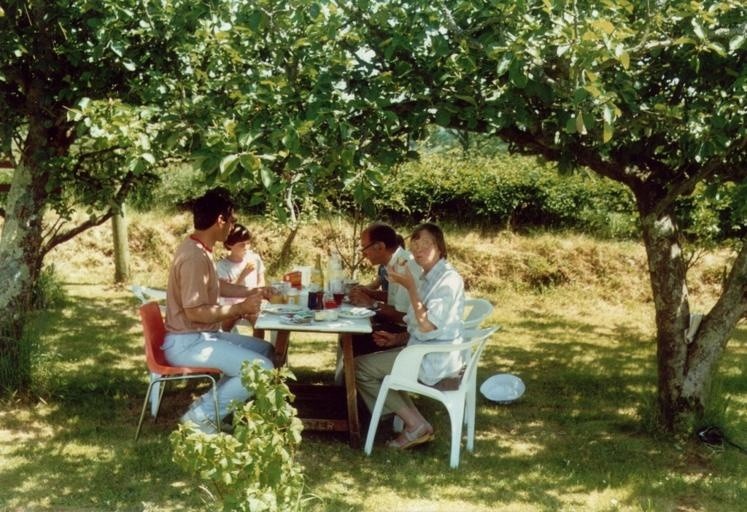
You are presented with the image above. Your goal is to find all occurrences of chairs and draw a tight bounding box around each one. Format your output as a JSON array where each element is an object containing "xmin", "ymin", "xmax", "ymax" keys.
[
  {"xmin": 363, "ymin": 324, "xmax": 501, "ymax": 470},
  {"xmin": 135, "ymin": 301, "xmax": 223, "ymax": 440},
  {"xmin": 456, "ymin": 299, "xmax": 495, "ymax": 367},
  {"xmin": 129, "ymin": 284, "xmax": 222, "ymax": 421}
]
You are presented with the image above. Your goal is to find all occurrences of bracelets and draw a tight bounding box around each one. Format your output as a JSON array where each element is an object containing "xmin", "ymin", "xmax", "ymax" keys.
[{"xmin": 398, "ymin": 332, "xmax": 408, "ymax": 345}]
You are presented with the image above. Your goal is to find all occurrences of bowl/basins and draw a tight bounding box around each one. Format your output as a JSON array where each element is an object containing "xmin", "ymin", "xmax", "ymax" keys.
[{"xmin": 271, "ymin": 281, "xmax": 291, "ymax": 293}]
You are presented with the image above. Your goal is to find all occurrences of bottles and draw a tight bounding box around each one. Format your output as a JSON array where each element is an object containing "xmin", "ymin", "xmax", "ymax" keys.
[
  {"xmin": 307, "ymin": 252, "xmax": 323, "ymax": 309},
  {"xmin": 327, "ymin": 250, "xmax": 343, "ymax": 297}
]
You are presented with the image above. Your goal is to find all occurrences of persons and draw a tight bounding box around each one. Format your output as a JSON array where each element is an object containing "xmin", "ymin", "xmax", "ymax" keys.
[
  {"xmin": 353, "ymin": 223, "xmax": 464, "ymax": 451},
  {"xmin": 347, "ymin": 224, "xmax": 423, "ymax": 442},
  {"xmin": 342, "ymin": 234, "xmax": 405, "ymax": 292},
  {"xmin": 216, "ymin": 223, "xmax": 290, "ymax": 368},
  {"xmin": 159, "ymin": 186, "xmax": 277, "ymax": 437}
]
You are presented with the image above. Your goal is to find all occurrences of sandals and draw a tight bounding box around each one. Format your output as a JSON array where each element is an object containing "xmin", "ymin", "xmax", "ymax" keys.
[{"xmin": 385, "ymin": 421, "xmax": 435, "ymax": 450}]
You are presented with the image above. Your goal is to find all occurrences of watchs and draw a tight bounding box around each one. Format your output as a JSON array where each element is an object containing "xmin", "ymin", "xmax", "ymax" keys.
[{"xmin": 371, "ymin": 300, "xmax": 384, "ymax": 309}]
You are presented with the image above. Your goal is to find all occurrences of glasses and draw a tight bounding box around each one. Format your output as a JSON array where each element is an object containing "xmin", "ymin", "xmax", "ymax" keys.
[{"xmin": 411, "ymin": 238, "xmax": 436, "ymax": 250}]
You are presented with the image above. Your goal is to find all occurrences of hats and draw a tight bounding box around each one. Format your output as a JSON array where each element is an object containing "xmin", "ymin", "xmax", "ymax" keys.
[{"xmin": 479, "ymin": 373, "xmax": 525, "ymax": 405}]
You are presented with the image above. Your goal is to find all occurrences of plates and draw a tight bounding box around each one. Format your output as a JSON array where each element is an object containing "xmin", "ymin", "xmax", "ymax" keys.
[
  {"xmin": 262, "ymin": 303, "xmax": 303, "ymax": 314},
  {"xmin": 332, "ymin": 307, "xmax": 374, "ymax": 319}
]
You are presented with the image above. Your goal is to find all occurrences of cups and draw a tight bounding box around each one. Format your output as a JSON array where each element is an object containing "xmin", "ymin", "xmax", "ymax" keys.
[
  {"xmin": 269, "ymin": 294, "xmax": 299, "ymax": 305},
  {"xmin": 282, "ymin": 271, "xmax": 302, "ymax": 290},
  {"xmin": 324, "ymin": 291, "xmax": 344, "ymax": 309},
  {"xmin": 313, "ymin": 311, "xmax": 325, "ymax": 322}
]
